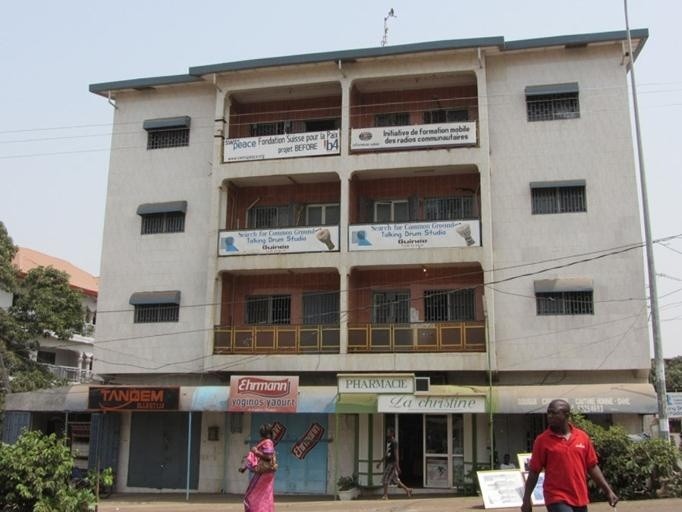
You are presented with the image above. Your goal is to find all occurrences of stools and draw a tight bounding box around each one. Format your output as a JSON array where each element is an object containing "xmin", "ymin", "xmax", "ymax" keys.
[{"xmin": 457, "ymin": 482, "xmax": 474, "ymax": 496}]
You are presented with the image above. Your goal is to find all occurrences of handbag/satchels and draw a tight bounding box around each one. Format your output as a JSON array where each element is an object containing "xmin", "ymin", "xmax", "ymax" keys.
[{"xmin": 247, "ymin": 452, "xmax": 278, "ymax": 474}]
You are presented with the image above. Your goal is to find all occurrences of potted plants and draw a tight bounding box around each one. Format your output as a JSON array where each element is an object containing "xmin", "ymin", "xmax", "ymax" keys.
[{"xmin": 335, "ymin": 475, "xmax": 361, "ymax": 501}]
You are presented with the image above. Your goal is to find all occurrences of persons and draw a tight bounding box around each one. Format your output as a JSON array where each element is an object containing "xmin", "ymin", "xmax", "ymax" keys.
[
  {"xmin": 376, "ymin": 427, "xmax": 413, "ymax": 500},
  {"xmin": 238, "ymin": 423, "xmax": 277, "ymax": 512},
  {"xmin": 520, "ymin": 399, "xmax": 619, "ymax": 512}
]
